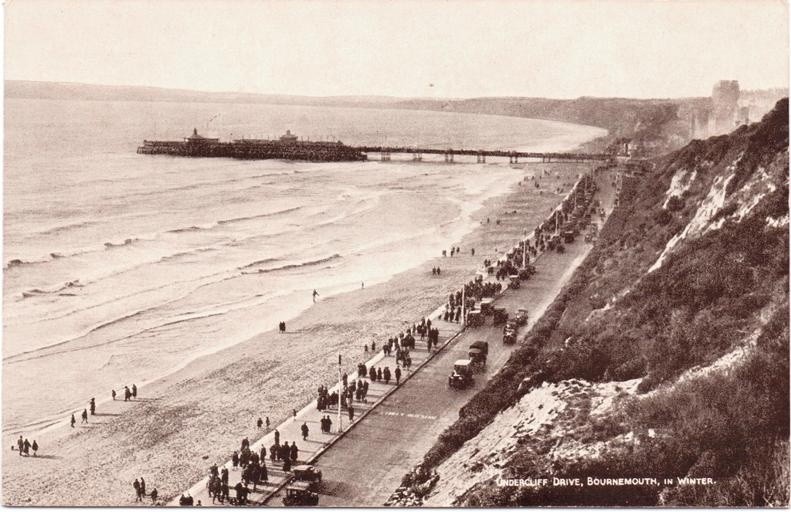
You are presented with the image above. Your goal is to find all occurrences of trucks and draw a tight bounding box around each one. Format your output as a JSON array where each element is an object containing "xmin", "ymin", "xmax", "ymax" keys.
[{"xmin": 468, "ymin": 340, "xmax": 488, "ymax": 374}]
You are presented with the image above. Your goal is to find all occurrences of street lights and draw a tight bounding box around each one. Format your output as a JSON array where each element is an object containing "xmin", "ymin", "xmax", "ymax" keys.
[{"xmin": 338, "ymin": 353, "xmax": 343, "ymax": 432}]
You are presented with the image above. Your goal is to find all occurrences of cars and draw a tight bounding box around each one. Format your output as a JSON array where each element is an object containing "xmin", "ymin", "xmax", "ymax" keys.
[
  {"xmin": 547, "ymin": 184, "xmax": 605, "ymax": 250},
  {"xmin": 510, "ymin": 265, "xmax": 536, "ymax": 289},
  {"xmin": 282, "ymin": 465, "xmax": 322, "ymax": 506},
  {"xmin": 467, "ymin": 299, "xmax": 528, "ymax": 344},
  {"xmin": 449, "ymin": 359, "xmax": 474, "ymax": 389}
]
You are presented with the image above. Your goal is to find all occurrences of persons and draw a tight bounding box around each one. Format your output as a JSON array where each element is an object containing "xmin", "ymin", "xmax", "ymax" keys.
[
  {"xmin": 148, "ymin": 486, "xmax": 158, "ymax": 504},
  {"xmin": 23, "ymin": 438, "xmax": 32, "ymax": 456},
  {"xmin": 179, "ymin": 318, "xmax": 442, "ymax": 507},
  {"xmin": 132, "ymin": 384, "xmax": 138, "ymax": 398},
  {"xmin": 81, "ymin": 408, "xmax": 89, "ymax": 424},
  {"xmin": 140, "ymin": 476, "xmax": 146, "ymax": 496},
  {"xmin": 125, "ymin": 385, "xmax": 131, "ymax": 401},
  {"xmin": 133, "ymin": 478, "xmax": 141, "ymax": 489},
  {"xmin": 17, "ymin": 435, "xmax": 24, "ymax": 455},
  {"xmin": 71, "ymin": 413, "xmax": 76, "ymax": 427},
  {"xmin": 136, "ymin": 486, "xmax": 144, "ymax": 502},
  {"xmin": 32, "ymin": 439, "xmax": 39, "ymax": 455},
  {"xmin": 90, "ymin": 397, "xmax": 96, "ymax": 414},
  {"xmin": 112, "ymin": 389, "xmax": 117, "ymax": 400},
  {"xmin": 278, "ymin": 288, "xmax": 319, "ymax": 333},
  {"xmin": 433, "ymin": 162, "xmax": 620, "ymax": 324}
]
